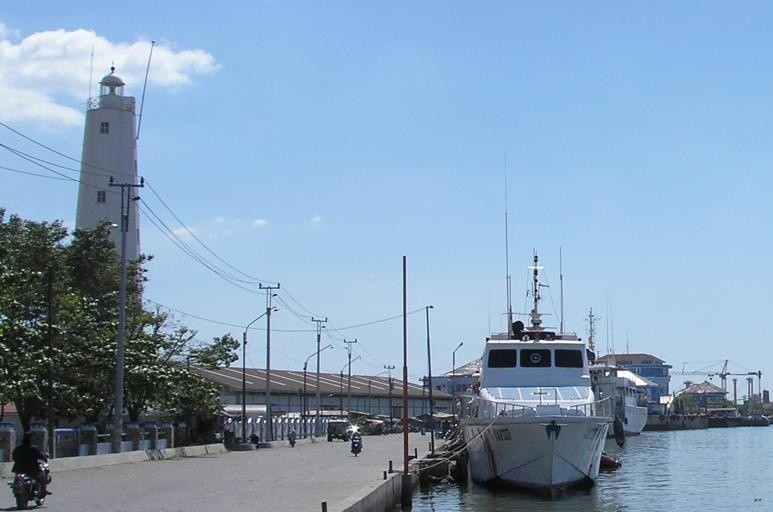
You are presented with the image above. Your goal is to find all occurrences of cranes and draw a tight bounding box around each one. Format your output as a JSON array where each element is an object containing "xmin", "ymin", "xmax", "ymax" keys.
[{"xmin": 668, "ymin": 360, "xmax": 762, "ymax": 393}]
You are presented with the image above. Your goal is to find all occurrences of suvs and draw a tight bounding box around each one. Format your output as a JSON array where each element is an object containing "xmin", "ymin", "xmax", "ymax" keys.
[{"xmin": 326, "ymin": 420, "xmax": 351, "ymax": 442}]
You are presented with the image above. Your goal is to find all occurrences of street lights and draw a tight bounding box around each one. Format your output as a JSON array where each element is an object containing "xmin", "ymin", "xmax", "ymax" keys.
[
  {"xmin": 47, "ymin": 220, "xmax": 118, "ymax": 460},
  {"xmin": 303, "ymin": 344, "xmax": 334, "ymax": 440},
  {"xmin": 452, "ymin": 342, "xmax": 463, "ymax": 375},
  {"xmin": 340, "ymin": 355, "xmax": 362, "ymax": 419},
  {"xmin": 241, "ymin": 306, "xmax": 279, "ymax": 446},
  {"xmin": 367, "ymin": 371, "xmax": 389, "ymax": 415}
]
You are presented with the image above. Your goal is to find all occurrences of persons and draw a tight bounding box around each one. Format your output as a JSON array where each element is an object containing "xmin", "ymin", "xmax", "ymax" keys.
[
  {"xmin": 351, "ymin": 429, "xmax": 362, "ymax": 452},
  {"xmin": 289, "ymin": 428, "xmax": 296, "ymax": 443},
  {"xmin": 249, "ymin": 431, "xmax": 260, "ymax": 449},
  {"xmin": 11, "ymin": 432, "xmax": 48, "ymax": 498}
]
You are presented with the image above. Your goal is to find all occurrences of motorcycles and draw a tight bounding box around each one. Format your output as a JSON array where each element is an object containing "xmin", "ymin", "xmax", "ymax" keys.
[
  {"xmin": 10, "ymin": 452, "xmax": 53, "ymax": 508},
  {"xmin": 287, "ymin": 431, "xmax": 296, "ymax": 448},
  {"xmin": 350, "ymin": 436, "xmax": 362, "ymax": 458}
]
